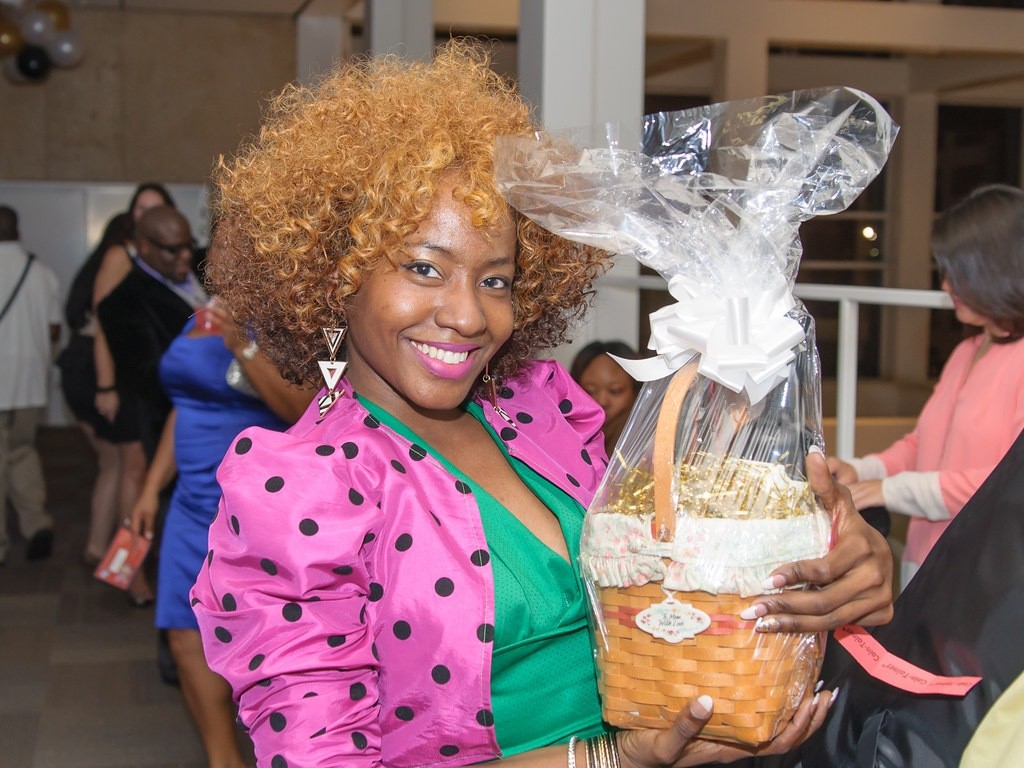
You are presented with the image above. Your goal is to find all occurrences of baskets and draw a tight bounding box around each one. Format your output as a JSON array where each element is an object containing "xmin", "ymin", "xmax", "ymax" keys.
[{"xmin": 580, "ymin": 348, "xmax": 836, "ymax": 748}]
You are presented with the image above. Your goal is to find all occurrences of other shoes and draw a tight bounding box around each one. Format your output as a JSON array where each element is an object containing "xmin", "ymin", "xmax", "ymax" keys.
[
  {"xmin": 126, "ymin": 589, "xmax": 156, "ymax": 608},
  {"xmin": 27, "ymin": 529, "xmax": 54, "ymax": 562}
]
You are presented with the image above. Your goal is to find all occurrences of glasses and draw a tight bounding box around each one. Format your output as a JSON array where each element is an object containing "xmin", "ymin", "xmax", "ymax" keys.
[{"xmin": 146, "ymin": 234, "xmax": 197, "ymax": 255}]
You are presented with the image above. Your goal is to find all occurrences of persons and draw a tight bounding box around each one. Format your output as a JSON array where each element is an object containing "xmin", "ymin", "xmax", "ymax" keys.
[
  {"xmin": 108, "ymin": 207, "xmax": 207, "ymax": 461},
  {"xmin": 566, "ymin": 339, "xmax": 638, "ymax": 456},
  {"xmin": 61, "ymin": 186, "xmax": 179, "ymax": 607},
  {"xmin": 192, "ymin": 34, "xmax": 895, "ymax": 768},
  {"xmin": 100, "ymin": 291, "xmax": 319, "ymax": 768},
  {"xmin": 0, "ymin": 204, "xmax": 62, "ymax": 565},
  {"xmin": 828, "ymin": 183, "xmax": 1023, "ymax": 598}
]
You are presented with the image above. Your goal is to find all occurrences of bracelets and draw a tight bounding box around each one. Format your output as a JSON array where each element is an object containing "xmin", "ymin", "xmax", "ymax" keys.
[
  {"xmin": 568, "ymin": 732, "xmax": 622, "ymax": 768},
  {"xmin": 96, "ymin": 384, "xmax": 116, "ymax": 393}
]
